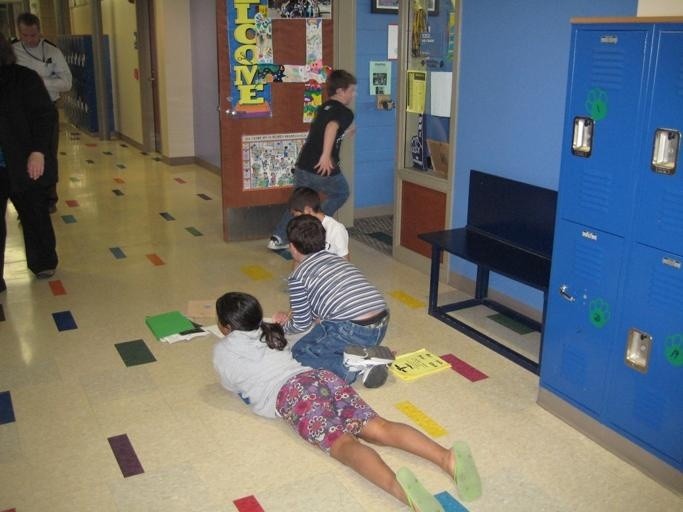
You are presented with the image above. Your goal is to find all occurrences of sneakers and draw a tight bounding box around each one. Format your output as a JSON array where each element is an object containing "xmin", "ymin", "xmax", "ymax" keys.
[
  {"xmin": 37, "ymin": 268, "xmax": 55, "ymax": 278},
  {"xmin": 344, "ymin": 343, "xmax": 397, "ymax": 388},
  {"xmin": 267, "ymin": 236, "xmax": 291, "ymax": 252}
]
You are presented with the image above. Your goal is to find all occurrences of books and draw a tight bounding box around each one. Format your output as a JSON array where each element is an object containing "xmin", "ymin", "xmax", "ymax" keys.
[{"xmin": 388, "ymin": 348, "xmax": 452, "ymax": 381}]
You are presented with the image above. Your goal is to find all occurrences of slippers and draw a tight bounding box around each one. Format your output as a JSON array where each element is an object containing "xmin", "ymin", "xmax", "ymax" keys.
[
  {"xmin": 451, "ymin": 440, "xmax": 483, "ymax": 502},
  {"xmin": 395, "ymin": 465, "xmax": 442, "ymax": 511}
]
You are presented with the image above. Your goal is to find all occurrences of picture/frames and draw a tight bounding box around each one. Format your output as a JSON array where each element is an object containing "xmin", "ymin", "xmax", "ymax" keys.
[{"xmin": 369, "ymin": 0, "xmax": 440, "ymax": 17}]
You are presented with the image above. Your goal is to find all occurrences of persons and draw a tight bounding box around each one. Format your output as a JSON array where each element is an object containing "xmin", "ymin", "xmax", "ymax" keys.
[
  {"xmin": 274, "ymin": 215, "xmax": 396, "ymax": 389},
  {"xmin": 1, "ymin": 37, "xmax": 61, "ymax": 291},
  {"xmin": 213, "ymin": 291, "xmax": 482, "ymax": 512},
  {"xmin": 266, "ymin": 69, "xmax": 357, "ymax": 250},
  {"xmin": 287, "ymin": 188, "xmax": 349, "ymax": 264},
  {"xmin": 9, "ymin": 13, "xmax": 73, "ymax": 218}
]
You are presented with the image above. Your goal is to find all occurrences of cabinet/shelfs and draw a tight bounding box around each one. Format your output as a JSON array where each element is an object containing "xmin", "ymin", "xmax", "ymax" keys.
[
  {"xmin": 557, "ymin": 16, "xmax": 653, "ymax": 242},
  {"xmin": 603, "ymin": 240, "xmax": 683, "ymax": 470},
  {"xmin": 541, "ymin": 218, "xmax": 628, "ymax": 428},
  {"xmin": 630, "ymin": 22, "xmax": 683, "ymax": 256},
  {"xmin": 56, "ymin": 33, "xmax": 116, "ymax": 139}
]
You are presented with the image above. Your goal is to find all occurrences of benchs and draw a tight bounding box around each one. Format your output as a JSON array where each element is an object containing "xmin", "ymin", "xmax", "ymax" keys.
[{"xmin": 417, "ymin": 170, "xmax": 558, "ymax": 373}]
